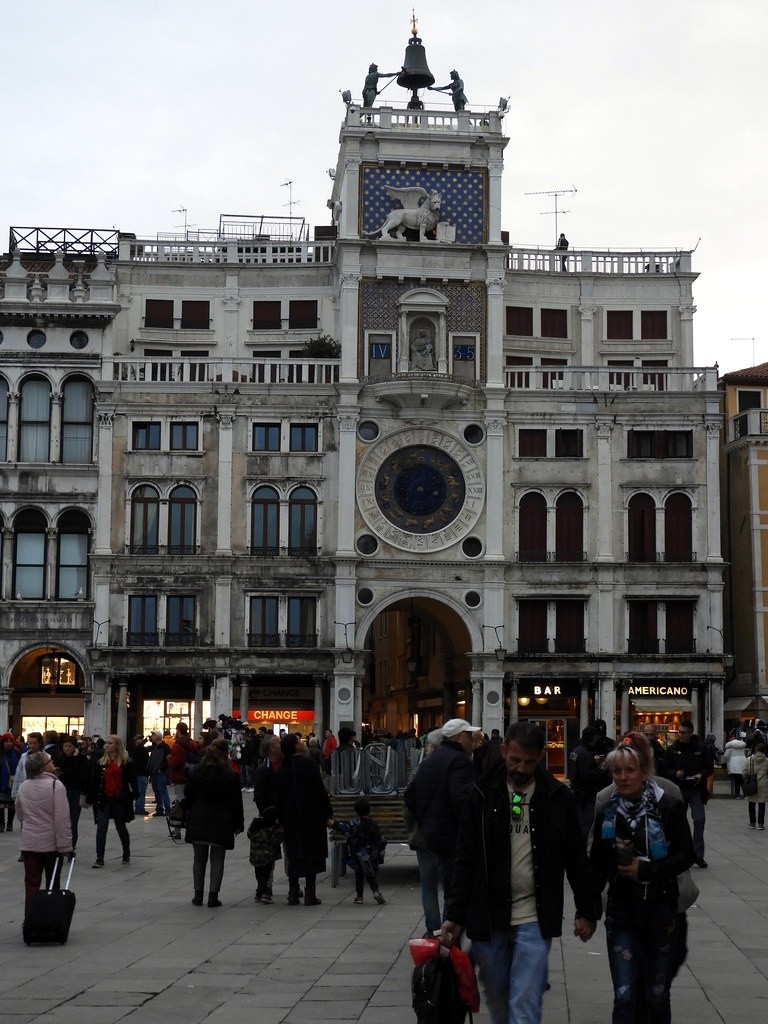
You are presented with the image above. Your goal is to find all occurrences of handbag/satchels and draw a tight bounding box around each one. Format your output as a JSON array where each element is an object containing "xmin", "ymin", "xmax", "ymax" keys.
[
  {"xmin": 79, "ymin": 794, "xmax": 89, "ymax": 809},
  {"xmin": 165, "ymin": 799, "xmax": 190, "ymax": 844},
  {"xmin": 743, "ymin": 757, "xmax": 758, "ymax": 796},
  {"xmin": 9, "ymin": 775, "xmax": 15, "ymax": 788},
  {"xmin": 678, "ymin": 871, "xmax": 699, "ymax": 914}
]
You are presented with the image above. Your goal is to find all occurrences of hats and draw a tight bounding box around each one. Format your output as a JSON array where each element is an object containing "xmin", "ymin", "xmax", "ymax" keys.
[
  {"xmin": 133, "ymin": 734, "xmax": 144, "ymax": 741},
  {"xmin": 0, "ymin": 733, "xmax": 14, "ymax": 744},
  {"xmin": 442, "ymin": 718, "xmax": 482, "ymax": 738},
  {"xmin": 151, "ymin": 731, "xmax": 162, "ymax": 739}
]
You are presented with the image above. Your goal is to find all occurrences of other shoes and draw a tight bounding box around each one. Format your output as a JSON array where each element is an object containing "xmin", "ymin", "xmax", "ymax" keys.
[
  {"xmin": 136, "ymin": 808, "xmax": 150, "ymax": 815},
  {"xmin": 92, "ymin": 858, "xmax": 104, "ymax": 868},
  {"xmin": 171, "ymin": 830, "xmax": 181, "ymax": 839},
  {"xmin": 758, "ymin": 824, "xmax": 764, "ymax": 829},
  {"xmin": 152, "ymin": 799, "xmax": 158, "ymax": 804},
  {"xmin": 695, "ymin": 858, "xmax": 708, "ymax": 868},
  {"xmin": 748, "ymin": 823, "xmax": 755, "ymax": 828},
  {"xmin": 68, "ymin": 853, "xmax": 73, "ymax": 863},
  {"xmin": 122, "ymin": 857, "xmax": 130, "ymax": 864}
]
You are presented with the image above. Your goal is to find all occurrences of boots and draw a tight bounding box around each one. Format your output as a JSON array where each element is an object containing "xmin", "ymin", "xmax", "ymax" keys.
[
  {"xmin": 7, "ymin": 808, "xmax": 15, "ymax": 831},
  {"xmin": 192, "ymin": 887, "xmax": 385, "ymax": 908},
  {"xmin": 165, "ymin": 807, "xmax": 171, "ymax": 816},
  {"xmin": 0, "ymin": 808, "xmax": 6, "ymax": 833},
  {"xmin": 152, "ymin": 807, "xmax": 164, "ymax": 817}
]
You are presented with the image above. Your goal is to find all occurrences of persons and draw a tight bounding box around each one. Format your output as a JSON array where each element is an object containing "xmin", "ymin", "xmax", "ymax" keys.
[
  {"xmin": 14, "ymin": 750, "xmax": 74, "ymax": 927},
  {"xmin": 185, "ymin": 738, "xmax": 244, "ymax": 907},
  {"xmin": 574, "ymin": 745, "xmax": 698, "ymax": 1024},
  {"xmin": 269, "ymin": 734, "xmax": 334, "ymax": 905},
  {"xmin": 360, "ymin": 63, "xmax": 401, "ymax": 126},
  {"xmin": 428, "ymin": 69, "xmax": 473, "ymax": 125},
  {"xmin": 247, "ymin": 806, "xmax": 287, "ymax": 904},
  {"xmin": 54, "ymin": 734, "xmax": 141, "ymax": 868},
  {"xmin": 438, "ymin": 722, "xmax": 602, "ymax": 1024},
  {"xmin": 0, "ymin": 715, "xmax": 768, "ymax": 833},
  {"xmin": 587, "ymin": 731, "xmax": 684, "ymax": 912},
  {"xmin": 403, "ymin": 718, "xmax": 493, "ymax": 941},
  {"xmin": 328, "ymin": 799, "xmax": 388, "ymax": 905},
  {"xmin": 554, "ymin": 233, "xmax": 569, "ymax": 272}
]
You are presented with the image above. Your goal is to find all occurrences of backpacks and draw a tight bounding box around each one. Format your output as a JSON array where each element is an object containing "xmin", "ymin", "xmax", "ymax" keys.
[
  {"xmin": 184, "ymin": 747, "xmax": 202, "ymax": 782},
  {"xmin": 410, "ymin": 936, "xmax": 480, "ymax": 1024}
]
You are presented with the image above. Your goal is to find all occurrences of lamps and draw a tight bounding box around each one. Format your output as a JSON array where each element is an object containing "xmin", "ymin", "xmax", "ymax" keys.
[
  {"xmin": 50, "ymin": 647, "xmax": 58, "ymax": 696},
  {"xmin": 483, "ymin": 625, "xmax": 507, "ymax": 661},
  {"xmin": 334, "ymin": 620, "xmax": 356, "ymax": 663},
  {"xmin": 89, "ymin": 619, "xmax": 110, "ymax": 660},
  {"xmin": 708, "ymin": 626, "xmax": 735, "ymax": 667}
]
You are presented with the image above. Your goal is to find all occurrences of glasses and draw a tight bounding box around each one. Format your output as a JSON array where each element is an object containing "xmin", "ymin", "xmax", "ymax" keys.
[
  {"xmin": 511, "ymin": 790, "xmax": 527, "ymax": 821},
  {"xmin": 41, "ymin": 758, "xmax": 51, "ymax": 767}
]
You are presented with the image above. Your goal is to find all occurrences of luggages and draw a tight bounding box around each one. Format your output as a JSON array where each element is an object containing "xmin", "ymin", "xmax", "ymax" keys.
[{"xmin": 22, "ymin": 852, "xmax": 76, "ymax": 946}]
